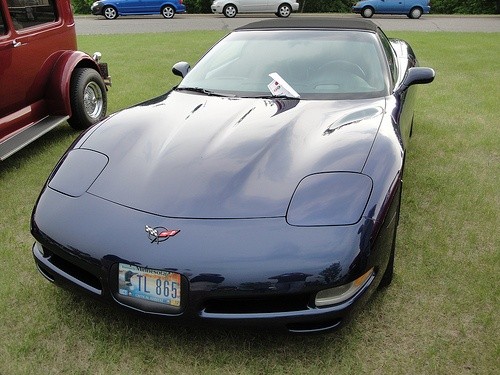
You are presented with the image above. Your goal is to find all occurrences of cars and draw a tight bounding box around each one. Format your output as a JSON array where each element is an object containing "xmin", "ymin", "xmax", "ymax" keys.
[
  {"xmin": 210, "ymin": 0, "xmax": 300, "ymax": 18},
  {"xmin": 90, "ymin": 0, "xmax": 186, "ymax": 20},
  {"xmin": 352, "ymin": 0, "xmax": 432, "ymax": 19}
]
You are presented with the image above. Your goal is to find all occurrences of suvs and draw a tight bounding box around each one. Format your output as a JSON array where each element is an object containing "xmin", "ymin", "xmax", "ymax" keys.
[{"xmin": 0, "ymin": 0, "xmax": 112, "ymax": 161}]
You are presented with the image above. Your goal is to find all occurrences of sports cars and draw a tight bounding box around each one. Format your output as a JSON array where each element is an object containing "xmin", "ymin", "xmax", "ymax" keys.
[{"xmin": 30, "ymin": 17, "xmax": 436, "ymax": 337}]
